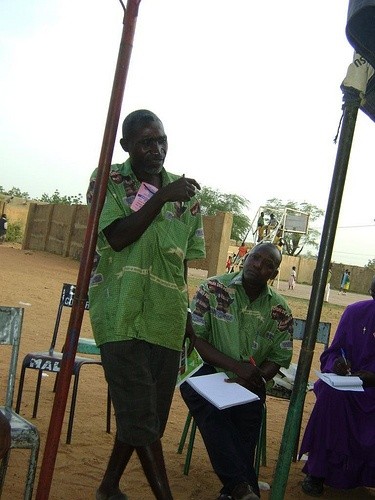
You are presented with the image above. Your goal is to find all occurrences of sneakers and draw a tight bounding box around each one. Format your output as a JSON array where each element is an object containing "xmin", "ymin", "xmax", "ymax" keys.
[
  {"xmin": 218, "ymin": 487, "xmax": 231, "ymax": 499},
  {"xmin": 232, "ymin": 482, "xmax": 258, "ymax": 500}
]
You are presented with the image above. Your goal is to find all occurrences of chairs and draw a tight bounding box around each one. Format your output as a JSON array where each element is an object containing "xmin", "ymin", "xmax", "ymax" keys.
[
  {"xmin": 177, "ymin": 404, "xmax": 265, "ymax": 479},
  {"xmin": 0, "ymin": 305, "xmax": 40, "ymax": 500},
  {"xmin": 265, "ymin": 318, "xmax": 331, "ymax": 463},
  {"xmin": 16, "ymin": 282, "xmax": 112, "ymax": 445}
]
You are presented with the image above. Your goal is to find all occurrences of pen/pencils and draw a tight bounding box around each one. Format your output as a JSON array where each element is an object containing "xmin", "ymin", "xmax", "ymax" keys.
[
  {"xmin": 181, "ymin": 174, "xmax": 185, "ymax": 208},
  {"xmin": 341, "ymin": 349, "xmax": 351, "ymax": 375},
  {"xmin": 250, "ymin": 356, "xmax": 266, "ymax": 384}
]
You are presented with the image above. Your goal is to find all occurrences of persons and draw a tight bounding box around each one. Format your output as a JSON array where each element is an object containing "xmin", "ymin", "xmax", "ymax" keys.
[
  {"xmin": 323, "ymin": 264, "xmax": 334, "ymax": 302},
  {"xmin": 86, "ymin": 110, "xmax": 207, "ymax": 500},
  {"xmin": 339, "ymin": 269, "xmax": 351, "ymax": 296},
  {"xmin": 0, "ymin": 213, "xmax": 9, "ymax": 242},
  {"xmin": 226, "ymin": 212, "xmax": 285, "ymax": 286},
  {"xmin": 288, "ymin": 266, "xmax": 296, "ymax": 291},
  {"xmin": 301, "ymin": 282, "xmax": 374, "ymax": 496},
  {"xmin": 179, "ymin": 241, "xmax": 290, "ymax": 499}
]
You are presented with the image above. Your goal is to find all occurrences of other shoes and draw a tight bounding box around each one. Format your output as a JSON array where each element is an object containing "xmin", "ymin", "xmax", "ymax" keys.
[{"xmin": 303, "ymin": 474, "xmax": 324, "ymax": 495}]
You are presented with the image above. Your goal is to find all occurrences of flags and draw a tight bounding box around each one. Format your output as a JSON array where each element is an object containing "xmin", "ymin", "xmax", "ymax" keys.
[{"xmin": 340, "ymin": 1, "xmax": 375, "ymax": 122}]
